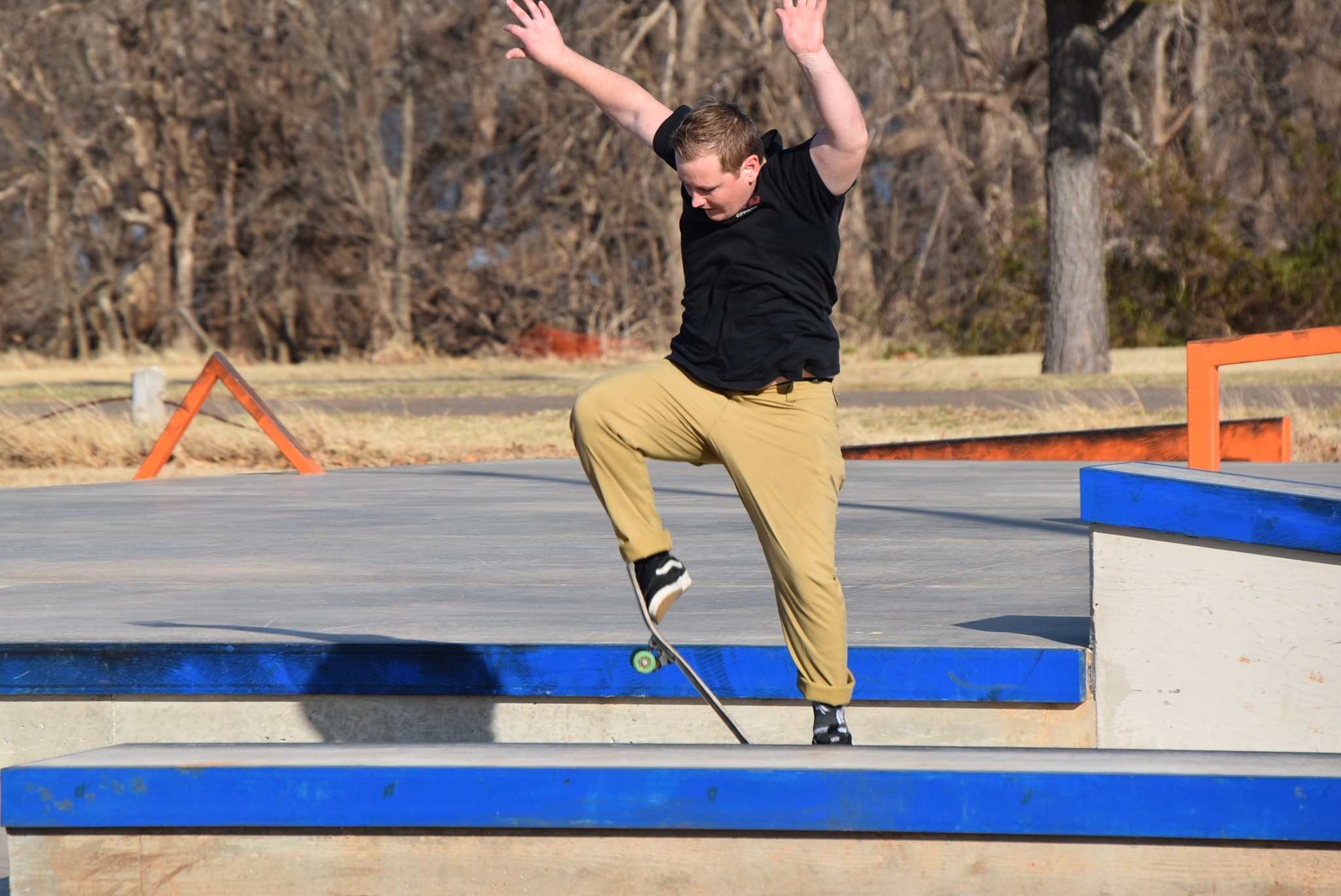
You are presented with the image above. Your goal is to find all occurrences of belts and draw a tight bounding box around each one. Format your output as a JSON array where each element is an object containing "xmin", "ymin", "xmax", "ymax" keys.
[{"xmin": 762, "ymin": 367, "xmax": 834, "ymax": 387}]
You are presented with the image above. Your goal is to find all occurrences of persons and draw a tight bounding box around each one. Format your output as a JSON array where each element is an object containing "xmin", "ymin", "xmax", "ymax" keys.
[{"xmin": 503, "ymin": 0, "xmax": 869, "ymax": 744}]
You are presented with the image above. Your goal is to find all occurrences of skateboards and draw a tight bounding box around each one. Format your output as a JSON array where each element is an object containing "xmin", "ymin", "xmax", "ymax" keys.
[{"xmin": 626, "ymin": 560, "xmax": 752, "ymax": 744}]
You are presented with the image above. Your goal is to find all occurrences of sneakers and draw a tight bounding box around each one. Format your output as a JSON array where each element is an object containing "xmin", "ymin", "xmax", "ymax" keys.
[
  {"xmin": 812, "ymin": 701, "xmax": 852, "ymax": 746},
  {"xmin": 635, "ymin": 550, "xmax": 692, "ymax": 625}
]
